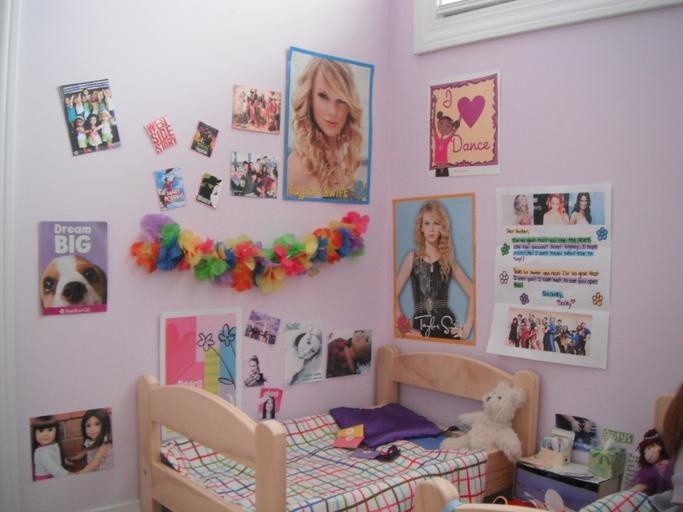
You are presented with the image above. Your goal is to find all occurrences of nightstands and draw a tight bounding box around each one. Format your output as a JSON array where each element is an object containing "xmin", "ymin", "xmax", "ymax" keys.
[{"xmin": 514, "ymin": 461, "xmax": 619, "ymax": 511}]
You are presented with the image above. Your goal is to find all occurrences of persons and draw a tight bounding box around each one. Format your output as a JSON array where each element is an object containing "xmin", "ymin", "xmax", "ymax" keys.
[
  {"xmin": 232, "ymin": 156, "xmax": 275, "ymax": 197},
  {"xmin": 287, "ymin": 56, "xmax": 365, "ymax": 197},
  {"xmin": 244, "ymin": 355, "xmax": 264, "ymax": 386},
  {"xmin": 260, "ymin": 396, "xmax": 277, "ymax": 418},
  {"xmin": 513, "ymin": 193, "xmax": 592, "ymax": 226},
  {"xmin": 63, "ymin": 88, "xmax": 117, "ymax": 154},
  {"xmin": 237, "ymin": 89, "xmax": 280, "ymax": 127},
  {"xmin": 396, "ymin": 201, "xmax": 474, "ymax": 340},
  {"xmin": 32, "ymin": 415, "xmax": 77, "ymax": 480},
  {"xmin": 160, "ymin": 176, "xmax": 177, "ymax": 193},
  {"xmin": 328, "ymin": 329, "xmax": 372, "ymax": 374},
  {"xmin": 509, "ymin": 313, "xmax": 591, "ymax": 356},
  {"xmin": 286, "ymin": 330, "xmax": 322, "ymax": 385},
  {"xmin": 430, "ymin": 94, "xmax": 460, "ymax": 177},
  {"xmin": 68, "ymin": 408, "xmax": 113, "ymax": 473}
]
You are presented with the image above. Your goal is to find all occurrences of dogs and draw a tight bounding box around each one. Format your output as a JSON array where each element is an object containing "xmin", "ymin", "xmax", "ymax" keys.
[{"xmin": 38, "ymin": 253, "xmax": 109, "ymax": 308}]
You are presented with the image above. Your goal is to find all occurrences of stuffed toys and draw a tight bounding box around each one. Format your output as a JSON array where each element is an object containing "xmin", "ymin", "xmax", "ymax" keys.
[
  {"xmin": 440, "ymin": 381, "xmax": 527, "ymax": 463},
  {"xmin": 625, "ymin": 428, "xmax": 673, "ymax": 494}
]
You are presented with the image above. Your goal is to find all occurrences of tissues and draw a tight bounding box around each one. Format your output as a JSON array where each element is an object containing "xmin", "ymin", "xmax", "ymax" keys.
[{"xmin": 587, "ymin": 434, "xmax": 626, "ymax": 478}]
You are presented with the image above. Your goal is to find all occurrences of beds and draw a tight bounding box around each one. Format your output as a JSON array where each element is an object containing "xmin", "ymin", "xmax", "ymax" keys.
[
  {"xmin": 413, "ymin": 394, "xmax": 683, "ymax": 512},
  {"xmin": 136, "ymin": 344, "xmax": 540, "ymax": 512}
]
